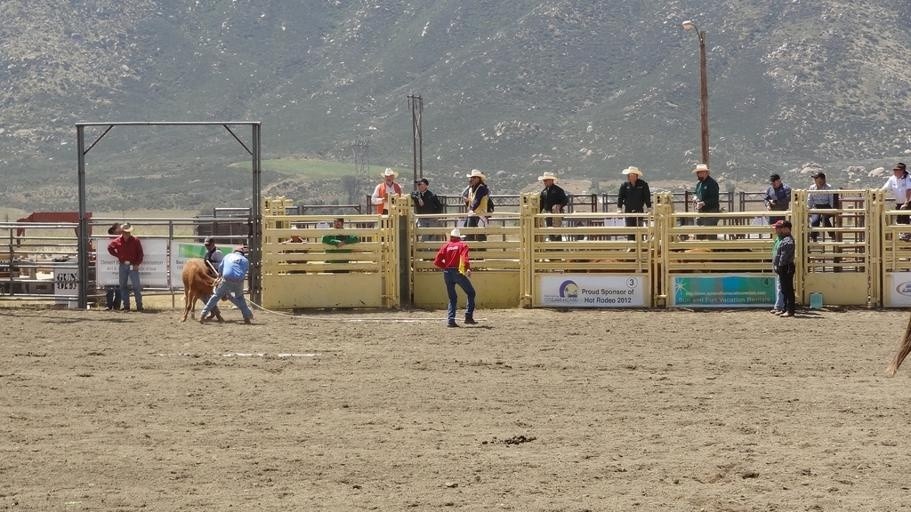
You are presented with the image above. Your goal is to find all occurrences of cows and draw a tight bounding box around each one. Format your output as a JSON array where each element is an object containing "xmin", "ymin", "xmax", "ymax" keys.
[{"xmin": 178, "ymin": 257, "xmax": 229, "ymax": 323}]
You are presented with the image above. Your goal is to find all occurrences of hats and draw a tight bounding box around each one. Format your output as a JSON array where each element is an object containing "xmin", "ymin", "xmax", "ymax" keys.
[
  {"xmin": 892, "ymin": 162, "xmax": 907, "ymax": 171},
  {"xmin": 380, "ymin": 168, "xmax": 399, "ymax": 180},
  {"xmin": 537, "ymin": 171, "xmax": 557, "ymax": 182},
  {"xmin": 810, "ymin": 172, "xmax": 826, "ymax": 179},
  {"xmin": 204, "ymin": 236, "xmax": 215, "ymax": 246},
  {"xmin": 692, "ymin": 163, "xmax": 712, "ymax": 173},
  {"xmin": 446, "ymin": 229, "xmax": 466, "ymax": 239},
  {"xmin": 466, "ymin": 168, "xmax": 486, "ymax": 181},
  {"xmin": 108, "ymin": 223, "xmax": 120, "ymax": 234},
  {"xmin": 771, "ymin": 219, "xmax": 792, "ymax": 229},
  {"xmin": 119, "ymin": 222, "xmax": 134, "ymax": 233},
  {"xmin": 414, "ymin": 178, "xmax": 429, "ymax": 186},
  {"xmin": 621, "ymin": 166, "xmax": 642, "ymax": 176}
]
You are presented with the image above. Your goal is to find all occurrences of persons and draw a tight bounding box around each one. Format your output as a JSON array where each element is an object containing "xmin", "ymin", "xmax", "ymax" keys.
[
  {"xmin": 690, "ymin": 164, "xmax": 719, "ymax": 240},
  {"xmin": 537, "ymin": 171, "xmax": 567, "ymax": 262},
  {"xmin": 371, "ymin": 168, "xmax": 494, "ymax": 269},
  {"xmin": 198, "ymin": 237, "xmax": 253, "ymax": 327},
  {"xmin": 806, "ymin": 172, "xmax": 835, "ymax": 242},
  {"xmin": 617, "ymin": 166, "xmax": 651, "ymax": 262},
  {"xmin": 106, "ymin": 221, "xmax": 145, "ymax": 312},
  {"xmin": 321, "ymin": 217, "xmax": 361, "ymax": 311},
  {"xmin": 879, "ymin": 162, "xmax": 911, "ymax": 240},
  {"xmin": 774, "ymin": 221, "xmax": 795, "ymax": 317},
  {"xmin": 434, "ymin": 228, "xmax": 479, "ymax": 327},
  {"xmin": 281, "ymin": 225, "xmax": 309, "ymax": 312},
  {"xmin": 770, "ymin": 219, "xmax": 784, "ymax": 313},
  {"xmin": 763, "ymin": 174, "xmax": 791, "ymax": 237}
]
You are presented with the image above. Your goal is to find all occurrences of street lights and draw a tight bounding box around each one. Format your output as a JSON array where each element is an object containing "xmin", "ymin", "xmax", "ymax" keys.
[{"xmin": 682, "ymin": 17, "xmax": 713, "ymax": 184}]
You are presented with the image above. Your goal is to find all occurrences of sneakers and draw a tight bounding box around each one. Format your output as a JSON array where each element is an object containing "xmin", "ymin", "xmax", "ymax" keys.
[
  {"xmin": 779, "ymin": 310, "xmax": 792, "ymax": 317},
  {"xmin": 770, "ymin": 309, "xmax": 780, "ymax": 313},
  {"xmin": 464, "ymin": 313, "xmax": 478, "ymax": 325},
  {"xmin": 775, "ymin": 310, "xmax": 785, "ymax": 315},
  {"xmin": 447, "ymin": 321, "xmax": 460, "ymax": 328}
]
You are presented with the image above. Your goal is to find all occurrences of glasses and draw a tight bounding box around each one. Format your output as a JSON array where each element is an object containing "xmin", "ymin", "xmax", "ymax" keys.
[{"xmin": 417, "ymin": 183, "xmax": 423, "ymax": 186}]
